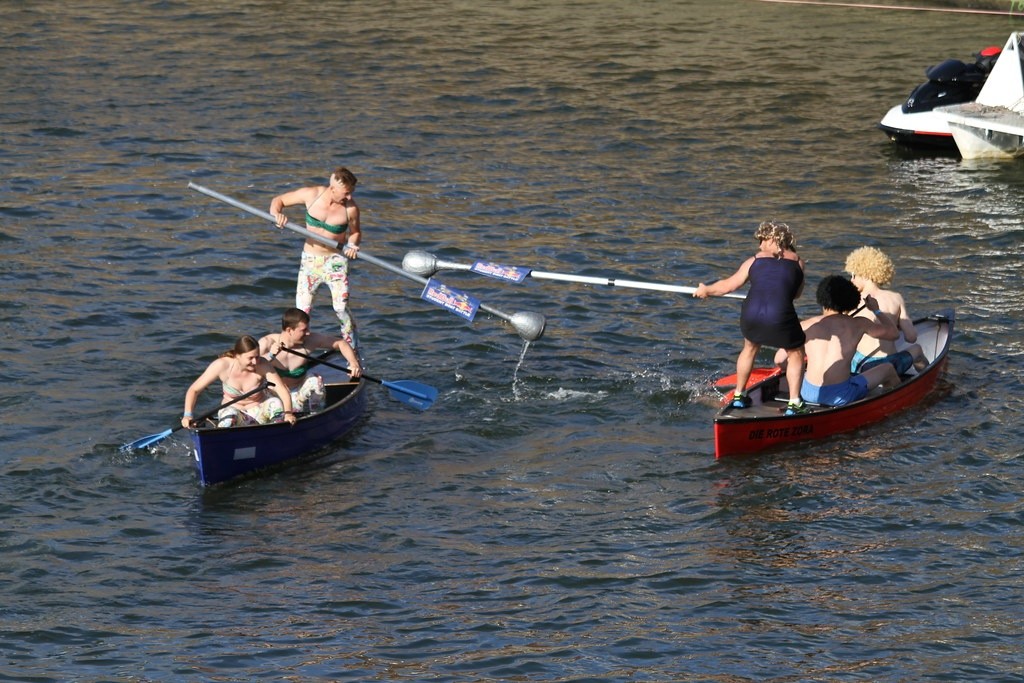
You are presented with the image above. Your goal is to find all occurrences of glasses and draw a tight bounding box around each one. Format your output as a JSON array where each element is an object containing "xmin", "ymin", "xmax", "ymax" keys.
[{"xmin": 760, "ymin": 238, "xmax": 768, "ymax": 244}]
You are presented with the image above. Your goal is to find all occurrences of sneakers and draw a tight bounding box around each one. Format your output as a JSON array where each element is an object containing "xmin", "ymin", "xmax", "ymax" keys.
[
  {"xmin": 785, "ymin": 401, "xmax": 814, "ymax": 415},
  {"xmin": 733, "ymin": 394, "xmax": 752, "ymax": 407}
]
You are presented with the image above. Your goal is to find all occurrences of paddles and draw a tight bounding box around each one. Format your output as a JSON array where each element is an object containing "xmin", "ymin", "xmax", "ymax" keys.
[
  {"xmin": 118, "ymin": 380, "xmax": 274, "ymax": 454},
  {"xmin": 716, "ymin": 296, "xmax": 869, "ymax": 404},
  {"xmin": 280, "ymin": 346, "xmax": 440, "ymax": 412}
]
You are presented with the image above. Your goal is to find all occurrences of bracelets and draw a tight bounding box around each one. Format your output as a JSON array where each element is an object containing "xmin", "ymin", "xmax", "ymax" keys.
[
  {"xmin": 875, "ymin": 310, "xmax": 880, "ymax": 314},
  {"xmin": 285, "ymin": 411, "xmax": 291, "ymax": 414},
  {"xmin": 269, "ymin": 352, "xmax": 275, "ymax": 359},
  {"xmin": 184, "ymin": 412, "xmax": 193, "ymax": 416}
]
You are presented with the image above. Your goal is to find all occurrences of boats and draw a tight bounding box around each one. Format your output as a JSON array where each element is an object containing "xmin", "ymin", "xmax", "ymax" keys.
[
  {"xmin": 878, "ymin": 31, "xmax": 1002, "ymax": 147},
  {"xmin": 713, "ymin": 307, "xmax": 956, "ymax": 458},
  {"xmin": 933, "ymin": 32, "xmax": 1024, "ymax": 159},
  {"xmin": 185, "ymin": 329, "xmax": 368, "ymax": 488}
]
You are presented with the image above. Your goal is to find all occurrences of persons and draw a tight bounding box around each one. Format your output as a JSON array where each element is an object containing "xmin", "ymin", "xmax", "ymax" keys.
[
  {"xmin": 270, "ymin": 168, "xmax": 361, "ymax": 369},
  {"xmin": 258, "ymin": 308, "xmax": 363, "ymax": 414},
  {"xmin": 845, "ymin": 246, "xmax": 929, "ymax": 375},
  {"xmin": 775, "ymin": 274, "xmax": 900, "ymax": 406},
  {"xmin": 693, "ymin": 221, "xmax": 811, "ymax": 415},
  {"xmin": 181, "ymin": 335, "xmax": 296, "ymax": 429}
]
